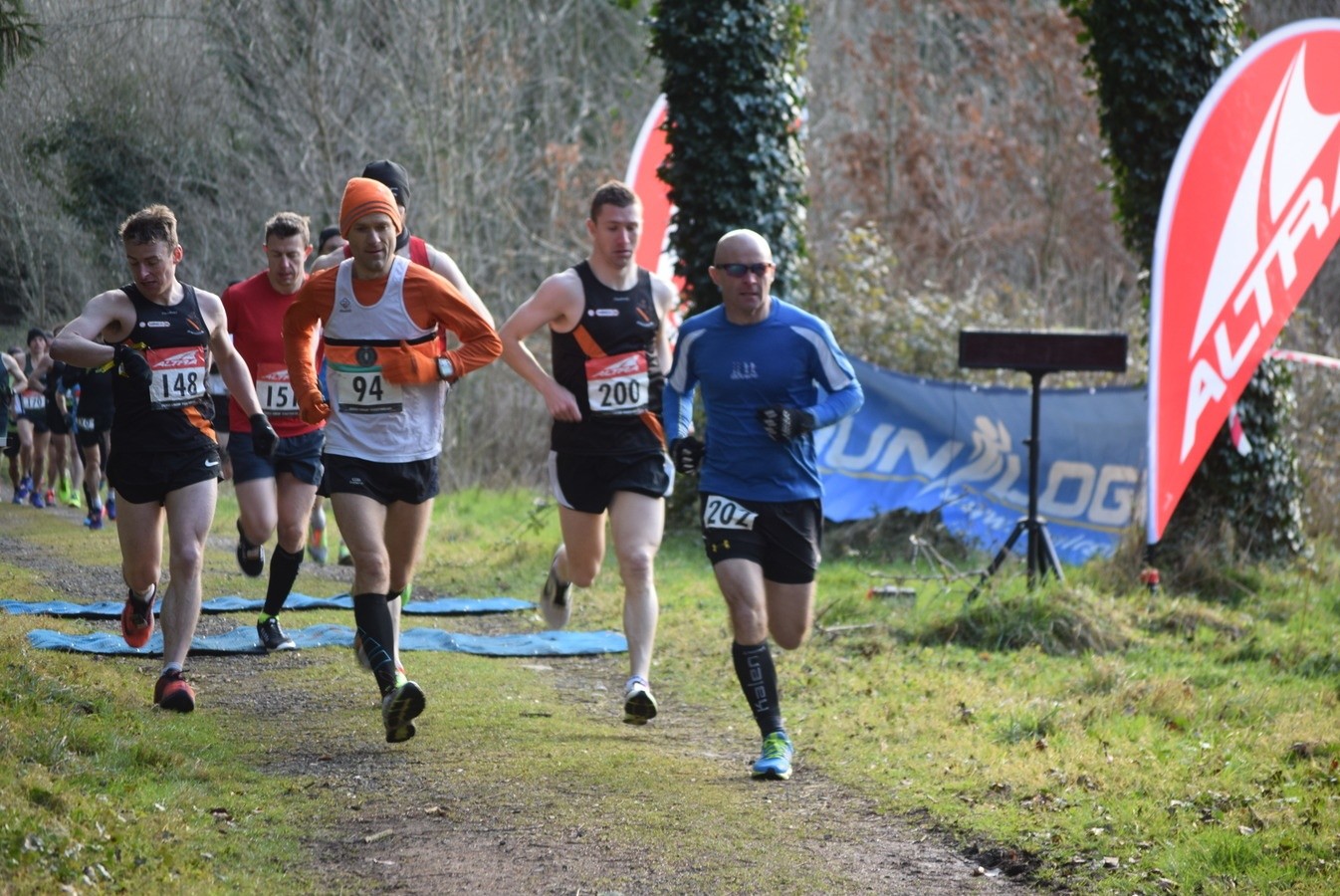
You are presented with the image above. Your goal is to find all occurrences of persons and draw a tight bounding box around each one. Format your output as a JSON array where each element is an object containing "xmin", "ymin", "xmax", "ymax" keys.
[
  {"xmin": 282, "ymin": 177, "xmax": 504, "ymax": 742},
  {"xmin": 496, "ymin": 182, "xmax": 682, "ymax": 725},
  {"xmin": 310, "ymin": 161, "xmax": 502, "ymax": 674},
  {"xmin": 49, "ymin": 205, "xmax": 279, "ymax": 713},
  {"xmin": 0, "ymin": 226, "xmax": 353, "ymax": 569},
  {"xmin": 663, "ymin": 228, "xmax": 865, "ymax": 782},
  {"xmin": 221, "ymin": 211, "xmax": 329, "ymax": 653}
]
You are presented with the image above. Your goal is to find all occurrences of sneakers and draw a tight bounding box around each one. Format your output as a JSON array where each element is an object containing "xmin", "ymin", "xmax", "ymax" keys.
[
  {"xmin": 381, "ymin": 681, "xmax": 426, "ymax": 743},
  {"xmin": 541, "ymin": 544, "xmax": 573, "ymax": 628},
  {"xmin": 121, "ymin": 582, "xmax": 155, "ymax": 649},
  {"xmin": 307, "ymin": 522, "xmax": 328, "ymax": 565},
  {"xmin": 236, "ymin": 518, "xmax": 265, "ymax": 578},
  {"xmin": 257, "ymin": 615, "xmax": 296, "ymax": 652},
  {"xmin": 153, "ymin": 667, "xmax": 197, "ymax": 713},
  {"xmin": 622, "ymin": 683, "xmax": 658, "ymax": 726},
  {"xmin": 12, "ymin": 475, "xmax": 116, "ymax": 530},
  {"xmin": 339, "ymin": 541, "xmax": 353, "ymax": 565},
  {"xmin": 748, "ymin": 730, "xmax": 792, "ymax": 780},
  {"xmin": 353, "ymin": 629, "xmax": 372, "ymax": 670}
]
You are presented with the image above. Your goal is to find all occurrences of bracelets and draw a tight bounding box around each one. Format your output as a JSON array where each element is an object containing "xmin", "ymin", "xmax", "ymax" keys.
[{"xmin": 12, "ymin": 388, "xmax": 17, "ymax": 396}]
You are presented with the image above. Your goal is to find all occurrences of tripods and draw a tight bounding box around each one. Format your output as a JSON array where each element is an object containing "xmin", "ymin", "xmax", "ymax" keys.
[{"xmin": 962, "ymin": 371, "xmax": 1069, "ymax": 607}]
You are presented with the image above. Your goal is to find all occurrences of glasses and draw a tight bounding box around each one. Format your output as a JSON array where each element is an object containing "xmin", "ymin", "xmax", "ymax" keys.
[{"xmin": 714, "ymin": 261, "xmax": 772, "ymax": 279}]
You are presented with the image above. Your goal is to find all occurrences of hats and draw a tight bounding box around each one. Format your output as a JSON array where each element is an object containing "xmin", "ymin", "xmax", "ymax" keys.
[
  {"xmin": 317, "ymin": 228, "xmax": 341, "ymax": 254},
  {"xmin": 27, "ymin": 328, "xmax": 48, "ymax": 347},
  {"xmin": 339, "ymin": 177, "xmax": 404, "ymax": 241},
  {"xmin": 361, "ymin": 158, "xmax": 410, "ymax": 213}
]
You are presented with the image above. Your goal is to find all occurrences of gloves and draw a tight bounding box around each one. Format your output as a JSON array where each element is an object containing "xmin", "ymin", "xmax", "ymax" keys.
[
  {"xmin": 299, "ymin": 388, "xmax": 331, "ymax": 425},
  {"xmin": 62, "ymin": 413, "xmax": 73, "ymax": 426},
  {"xmin": 113, "ymin": 345, "xmax": 154, "ymax": 389},
  {"xmin": 382, "ymin": 340, "xmax": 440, "ymax": 386},
  {"xmin": 248, "ymin": 414, "xmax": 280, "ymax": 461}
]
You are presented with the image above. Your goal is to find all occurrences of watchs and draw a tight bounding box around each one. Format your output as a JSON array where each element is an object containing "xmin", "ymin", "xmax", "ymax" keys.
[
  {"xmin": 113, "ymin": 343, "xmax": 128, "ymax": 366},
  {"xmin": 436, "ymin": 357, "xmax": 455, "ymax": 379}
]
